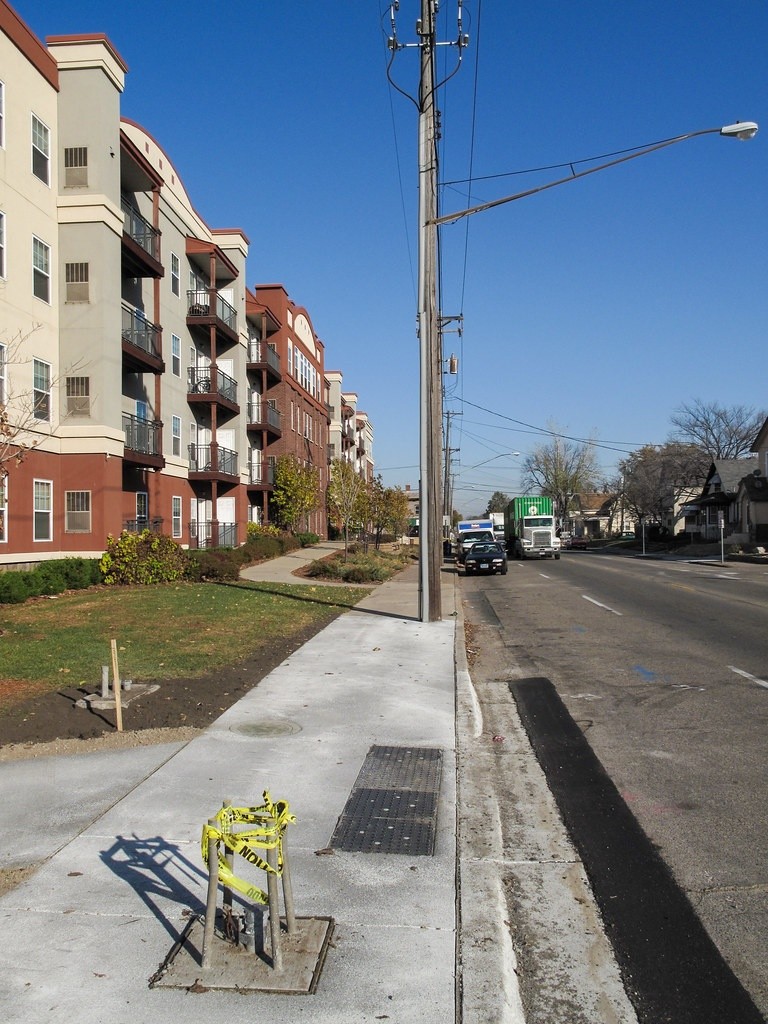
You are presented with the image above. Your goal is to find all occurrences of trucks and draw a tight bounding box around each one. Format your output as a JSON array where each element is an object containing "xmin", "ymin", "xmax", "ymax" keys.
[
  {"xmin": 489, "ymin": 512, "xmax": 504, "ymax": 538},
  {"xmin": 504, "ymin": 497, "xmax": 562, "ymax": 561}
]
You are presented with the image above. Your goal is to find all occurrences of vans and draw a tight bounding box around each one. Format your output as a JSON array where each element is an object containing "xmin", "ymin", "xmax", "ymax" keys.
[{"xmin": 456, "ymin": 531, "xmax": 494, "ymax": 564}]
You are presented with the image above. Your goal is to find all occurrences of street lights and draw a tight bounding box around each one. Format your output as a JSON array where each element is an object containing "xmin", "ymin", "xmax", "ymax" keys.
[
  {"xmin": 420, "ymin": 121, "xmax": 759, "ymax": 622},
  {"xmin": 450, "ymin": 486, "xmax": 474, "ymax": 530},
  {"xmin": 449, "ymin": 452, "xmax": 519, "ymax": 528}
]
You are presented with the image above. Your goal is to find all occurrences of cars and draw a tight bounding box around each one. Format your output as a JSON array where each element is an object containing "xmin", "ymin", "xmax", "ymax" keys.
[
  {"xmin": 566, "ymin": 537, "xmax": 587, "ymax": 550},
  {"xmin": 618, "ymin": 532, "xmax": 635, "ymax": 540},
  {"xmin": 464, "ymin": 541, "xmax": 508, "ymax": 576},
  {"xmin": 495, "ymin": 534, "xmax": 507, "ymax": 546}
]
